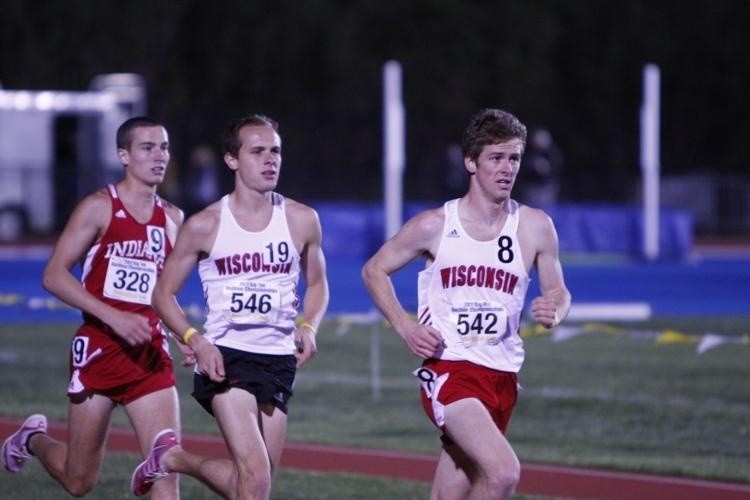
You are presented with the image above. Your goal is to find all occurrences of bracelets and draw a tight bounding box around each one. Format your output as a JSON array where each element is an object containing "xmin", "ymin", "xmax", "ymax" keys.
[
  {"xmin": 184, "ymin": 327, "xmax": 198, "ymax": 345},
  {"xmin": 298, "ymin": 322, "xmax": 318, "ymax": 335}
]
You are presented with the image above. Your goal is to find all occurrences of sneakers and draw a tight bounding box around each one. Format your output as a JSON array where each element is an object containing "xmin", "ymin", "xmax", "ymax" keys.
[
  {"xmin": 130, "ymin": 428, "xmax": 181, "ymax": 497},
  {"xmin": 1, "ymin": 414, "xmax": 47, "ymax": 473}
]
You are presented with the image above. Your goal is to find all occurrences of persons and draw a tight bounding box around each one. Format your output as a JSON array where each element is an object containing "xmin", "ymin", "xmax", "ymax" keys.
[
  {"xmin": 521, "ymin": 128, "xmax": 562, "ymax": 205},
  {"xmin": 361, "ymin": 108, "xmax": 573, "ymax": 500},
  {"xmin": 0, "ymin": 115, "xmax": 200, "ymax": 500},
  {"xmin": 129, "ymin": 118, "xmax": 328, "ymax": 500}
]
